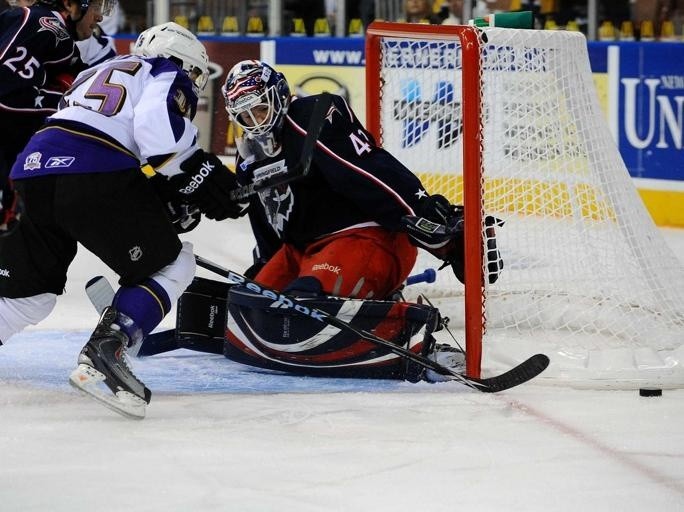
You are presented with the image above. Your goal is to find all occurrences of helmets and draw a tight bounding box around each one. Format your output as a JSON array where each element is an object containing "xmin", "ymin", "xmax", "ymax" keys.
[
  {"xmin": 133, "ymin": 20, "xmax": 211, "ymax": 94},
  {"xmin": 219, "ymin": 58, "xmax": 293, "ymax": 123}
]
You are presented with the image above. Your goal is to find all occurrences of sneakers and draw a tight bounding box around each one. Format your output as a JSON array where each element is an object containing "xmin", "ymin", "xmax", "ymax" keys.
[
  {"xmin": 424, "ymin": 339, "xmax": 467, "ymax": 382},
  {"xmin": 76, "ymin": 322, "xmax": 154, "ymax": 406}
]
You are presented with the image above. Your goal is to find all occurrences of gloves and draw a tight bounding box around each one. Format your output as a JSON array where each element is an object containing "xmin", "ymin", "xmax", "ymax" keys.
[
  {"xmin": 164, "ymin": 146, "xmax": 250, "ymax": 235},
  {"xmin": 402, "ymin": 193, "xmax": 506, "ymax": 288}
]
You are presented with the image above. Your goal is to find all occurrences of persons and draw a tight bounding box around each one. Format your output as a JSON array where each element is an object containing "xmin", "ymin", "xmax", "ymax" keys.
[
  {"xmin": 0, "ymin": 21, "xmax": 252, "ymax": 406},
  {"xmin": 220, "ymin": 60, "xmax": 503, "ymax": 382},
  {"xmin": 0, "ymin": 0, "xmax": 114, "ymax": 235}
]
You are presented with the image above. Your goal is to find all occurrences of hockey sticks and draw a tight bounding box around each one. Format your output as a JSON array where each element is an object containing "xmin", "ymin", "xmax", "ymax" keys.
[
  {"xmin": 85, "ymin": 276, "xmax": 182, "ymax": 358},
  {"xmin": 191, "ymin": 252, "xmax": 549, "ymax": 393},
  {"xmin": 178, "ymin": 92, "xmax": 333, "ymax": 216}
]
